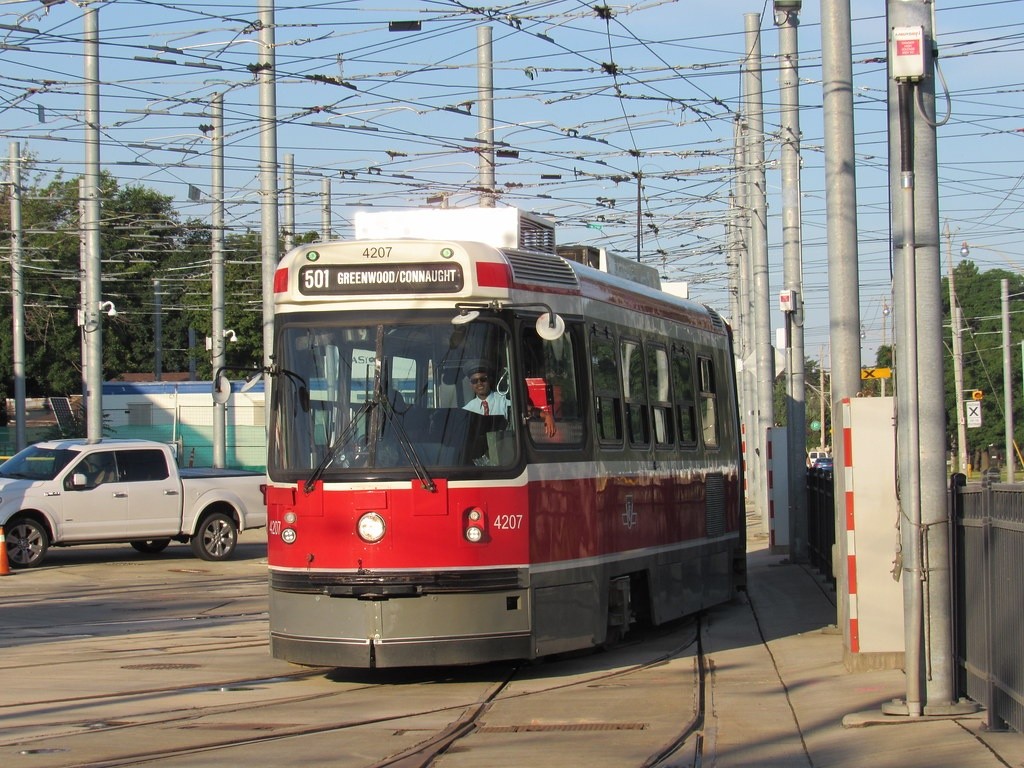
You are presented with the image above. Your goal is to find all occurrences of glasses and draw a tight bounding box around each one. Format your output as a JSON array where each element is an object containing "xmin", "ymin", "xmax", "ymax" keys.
[{"xmin": 470, "ymin": 376, "xmax": 488, "ymax": 384}]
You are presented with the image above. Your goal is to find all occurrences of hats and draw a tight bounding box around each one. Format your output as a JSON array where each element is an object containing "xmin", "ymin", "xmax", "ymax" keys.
[{"xmin": 462, "ymin": 359, "xmax": 492, "ymax": 379}]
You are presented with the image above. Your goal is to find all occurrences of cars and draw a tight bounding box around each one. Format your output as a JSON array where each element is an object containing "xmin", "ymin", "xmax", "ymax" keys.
[{"xmin": 814, "ymin": 458, "xmax": 832, "ymax": 473}]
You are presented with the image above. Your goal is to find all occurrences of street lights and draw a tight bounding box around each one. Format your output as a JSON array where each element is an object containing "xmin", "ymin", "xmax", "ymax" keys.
[{"xmin": 881, "ymin": 304, "xmax": 890, "ymax": 397}]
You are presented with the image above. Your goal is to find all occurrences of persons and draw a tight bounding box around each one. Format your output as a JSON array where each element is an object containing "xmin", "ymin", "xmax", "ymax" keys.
[
  {"xmin": 461, "ymin": 359, "xmax": 556, "ymax": 438},
  {"xmin": 66, "ymin": 461, "xmax": 89, "ymax": 489}
]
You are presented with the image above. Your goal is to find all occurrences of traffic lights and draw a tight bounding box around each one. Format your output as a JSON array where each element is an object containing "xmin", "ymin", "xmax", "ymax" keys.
[{"xmin": 974, "ymin": 391, "xmax": 983, "ymax": 401}]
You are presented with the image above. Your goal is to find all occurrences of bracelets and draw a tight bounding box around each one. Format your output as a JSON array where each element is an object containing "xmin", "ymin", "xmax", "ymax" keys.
[{"xmin": 530, "ymin": 407, "xmax": 541, "ymax": 418}]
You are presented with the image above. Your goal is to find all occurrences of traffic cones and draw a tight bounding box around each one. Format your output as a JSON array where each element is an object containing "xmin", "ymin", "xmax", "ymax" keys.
[{"xmin": 0, "ymin": 526, "xmax": 16, "ymax": 576}]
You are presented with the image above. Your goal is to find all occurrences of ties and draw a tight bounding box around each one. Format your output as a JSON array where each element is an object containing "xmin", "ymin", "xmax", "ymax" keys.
[{"xmin": 482, "ymin": 401, "xmax": 489, "ymax": 416}]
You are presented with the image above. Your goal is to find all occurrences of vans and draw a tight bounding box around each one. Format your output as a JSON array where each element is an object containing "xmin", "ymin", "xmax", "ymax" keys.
[{"xmin": 805, "ymin": 452, "xmax": 828, "ymax": 469}]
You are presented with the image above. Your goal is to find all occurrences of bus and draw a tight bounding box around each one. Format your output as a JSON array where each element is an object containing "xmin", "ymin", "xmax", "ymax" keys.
[{"xmin": 210, "ymin": 208, "xmax": 746, "ymax": 668}]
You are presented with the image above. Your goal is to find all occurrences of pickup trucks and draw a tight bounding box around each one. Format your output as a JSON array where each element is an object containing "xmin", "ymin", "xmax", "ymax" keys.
[{"xmin": 0, "ymin": 438, "xmax": 267, "ymax": 569}]
